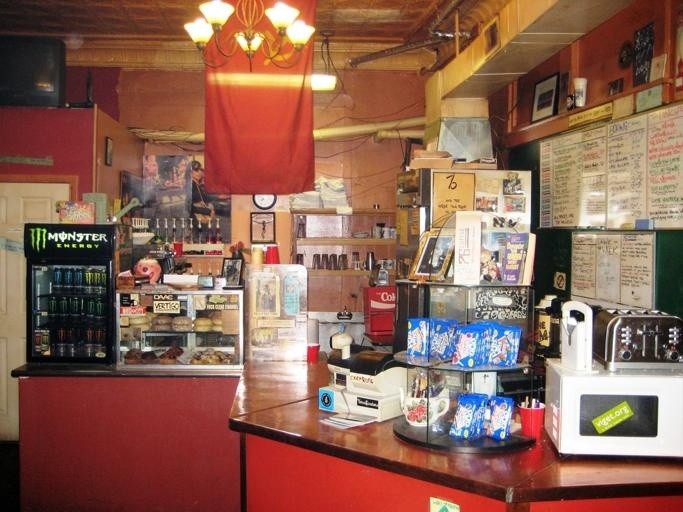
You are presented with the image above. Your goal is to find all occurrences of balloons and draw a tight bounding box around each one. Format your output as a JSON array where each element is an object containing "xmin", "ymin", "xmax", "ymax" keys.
[{"xmin": 251, "ymin": 194, "xmax": 277, "ymax": 211}]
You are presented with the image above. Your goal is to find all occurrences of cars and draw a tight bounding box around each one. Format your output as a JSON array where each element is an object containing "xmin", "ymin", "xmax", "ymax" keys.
[
  {"xmin": 518, "ymin": 402, "xmax": 544, "ymax": 437},
  {"xmin": 397, "ymin": 384, "xmax": 448, "ymax": 427},
  {"xmin": 573, "ymin": 77, "xmax": 587, "ymax": 108},
  {"xmin": 296, "ymin": 251, "xmax": 393, "ymax": 271},
  {"xmin": 266, "ymin": 246, "xmax": 279, "ymax": 264},
  {"xmin": 373, "ymin": 222, "xmax": 396, "ymax": 239},
  {"xmin": 306, "ymin": 343, "xmax": 320, "ymax": 363},
  {"xmin": 248, "ymin": 246, "xmax": 264, "ymax": 266}
]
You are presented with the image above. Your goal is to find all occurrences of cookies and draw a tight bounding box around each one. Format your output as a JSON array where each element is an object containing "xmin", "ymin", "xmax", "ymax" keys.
[{"xmin": 545, "ymin": 359, "xmax": 682, "ymax": 463}]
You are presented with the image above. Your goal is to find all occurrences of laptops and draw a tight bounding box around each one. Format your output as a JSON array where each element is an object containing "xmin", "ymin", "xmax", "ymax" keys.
[
  {"xmin": 128, "ymin": 312, "xmax": 222, "ymax": 332},
  {"xmin": 125, "ymin": 346, "xmax": 233, "ymax": 365}
]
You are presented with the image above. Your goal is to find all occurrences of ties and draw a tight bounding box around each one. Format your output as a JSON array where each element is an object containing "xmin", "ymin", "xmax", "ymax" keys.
[{"xmin": 0, "ymin": 34, "xmax": 63, "ymax": 109}]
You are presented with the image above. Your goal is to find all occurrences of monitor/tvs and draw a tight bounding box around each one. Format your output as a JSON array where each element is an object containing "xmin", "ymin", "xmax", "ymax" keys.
[{"xmin": 500, "ymin": 231, "xmax": 537, "ymax": 287}]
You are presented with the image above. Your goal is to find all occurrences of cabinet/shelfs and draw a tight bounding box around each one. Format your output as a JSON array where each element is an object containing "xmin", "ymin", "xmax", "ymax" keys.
[{"xmin": 296, "ymin": 223, "xmax": 305, "ymax": 238}]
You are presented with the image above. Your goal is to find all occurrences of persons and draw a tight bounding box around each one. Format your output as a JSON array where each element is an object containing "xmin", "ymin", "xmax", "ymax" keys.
[{"xmin": 182, "ymin": 160, "xmax": 220, "ymax": 244}]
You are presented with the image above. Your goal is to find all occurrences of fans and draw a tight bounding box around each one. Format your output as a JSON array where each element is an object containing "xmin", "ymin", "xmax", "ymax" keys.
[
  {"xmin": 538, "ymin": 100, "xmax": 683, "ymax": 230},
  {"xmin": 570, "ymin": 231, "xmax": 656, "ymax": 310}
]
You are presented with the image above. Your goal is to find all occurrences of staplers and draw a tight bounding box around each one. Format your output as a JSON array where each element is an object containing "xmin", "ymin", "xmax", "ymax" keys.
[
  {"xmin": 635, "ymin": 219, "xmax": 654, "ymax": 230},
  {"xmin": 622, "ymin": 223, "xmax": 633, "ymax": 229}
]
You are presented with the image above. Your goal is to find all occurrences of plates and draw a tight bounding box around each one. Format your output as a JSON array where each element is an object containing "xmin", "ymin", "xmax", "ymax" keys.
[
  {"xmin": 112, "ymin": 287, "xmax": 246, "ymax": 374},
  {"xmin": 286, "ymin": 205, "xmax": 397, "ymax": 280},
  {"xmin": 301, "ymin": 272, "xmax": 398, "ymax": 312}
]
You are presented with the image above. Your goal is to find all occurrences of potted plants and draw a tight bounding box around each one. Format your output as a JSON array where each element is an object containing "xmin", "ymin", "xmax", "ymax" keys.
[
  {"xmin": 407, "ymin": 225, "xmax": 459, "ymax": 283},
  {"xmin": 529, "ymin": 70, "xmax": 560, "ymax": 125},
  {"xmin": 104, "ymin": 134, "xmax": 113, "ymax": 167},
  {"xmin": 220, "ymin": 256, "xmax": 243, "ymax": 288},
  {"xmin": 248, "ymin": 212, "xmax": 275, "ymax": 245}
]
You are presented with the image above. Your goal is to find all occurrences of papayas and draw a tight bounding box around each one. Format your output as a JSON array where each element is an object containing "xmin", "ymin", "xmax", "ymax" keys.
[{"xmin": 595, "ymin": 307, "xmax": 683, "ymax": 370}]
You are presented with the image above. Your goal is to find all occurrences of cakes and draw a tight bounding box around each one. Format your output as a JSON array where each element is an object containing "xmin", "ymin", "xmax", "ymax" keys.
[{"xmin": 48, "ymin": 268, "xmax": 108, "ymax": 358}]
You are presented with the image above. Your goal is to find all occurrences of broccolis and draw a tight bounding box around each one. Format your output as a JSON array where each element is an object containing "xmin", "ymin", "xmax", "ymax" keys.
[{"xmin": 20, "ymin": 223, "xmax": 132, "ymax": 369}]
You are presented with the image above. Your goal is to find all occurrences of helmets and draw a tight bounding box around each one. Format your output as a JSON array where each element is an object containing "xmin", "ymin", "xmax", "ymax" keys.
[{"xmin": 353, "ymin": 231, "xmax": 367, "ymax": 238}]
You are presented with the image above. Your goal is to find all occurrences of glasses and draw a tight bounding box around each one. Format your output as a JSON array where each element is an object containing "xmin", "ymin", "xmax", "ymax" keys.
[
  {"xmin": 205, "ymin": 27, "xmax": 340, "ymax": 95},
  {"xmin": 182, "ymin": 0, "xmax": 318, "ymax": 77}
]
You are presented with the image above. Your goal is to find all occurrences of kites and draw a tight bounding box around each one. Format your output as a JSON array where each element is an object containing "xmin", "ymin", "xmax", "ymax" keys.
[{"xmin": 574, "ymin": 226, "xmax": 607, "ymax": 229}]
